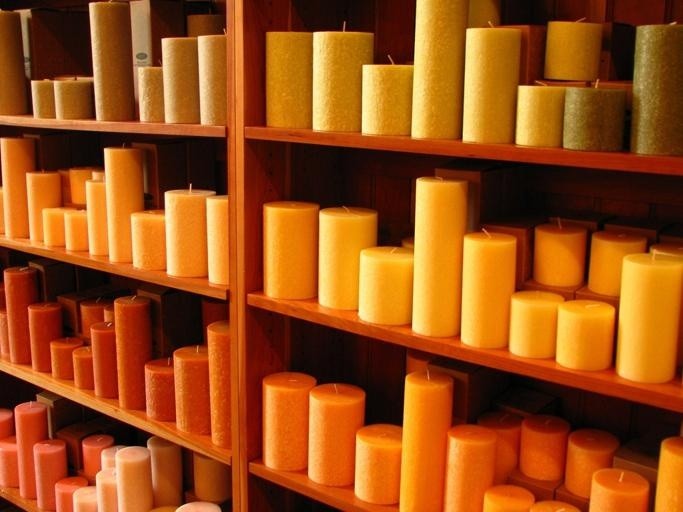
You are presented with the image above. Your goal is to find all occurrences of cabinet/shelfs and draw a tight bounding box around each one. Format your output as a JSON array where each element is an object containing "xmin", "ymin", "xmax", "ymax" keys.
[
  {"xmin": 1, "ymin": 0, "xmax": 241, "ymax": 510},
  {"xmin": 235, "ymin": 1, "xmax": 683, "ymax": 511}
]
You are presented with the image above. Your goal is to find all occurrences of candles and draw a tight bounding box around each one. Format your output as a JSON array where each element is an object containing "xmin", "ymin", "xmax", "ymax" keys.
[
  {"xmin": 261, "ymin": 168, "xmax": 682, "ymax": 386},
  {"xmin": 1, "ymin": 398, "xmax": 232, "ymax": 511},
  {"xmin": 0, "ymin": 3, "xmax": 228, "ymax": 124},
  {"xmin": 1, "ymin": 132, "xmax": 229, "ymax": 286},
  {"xmin": 268, "ymin": 2, "xmax": 682, "ymax": 155},
  {"xmin": 0, "ymin": 259, "xmax": 232, "ymax": 452},
  {"xmin": 259, "ymin": 347, "xmax": 681, "ymax": 512}
]
